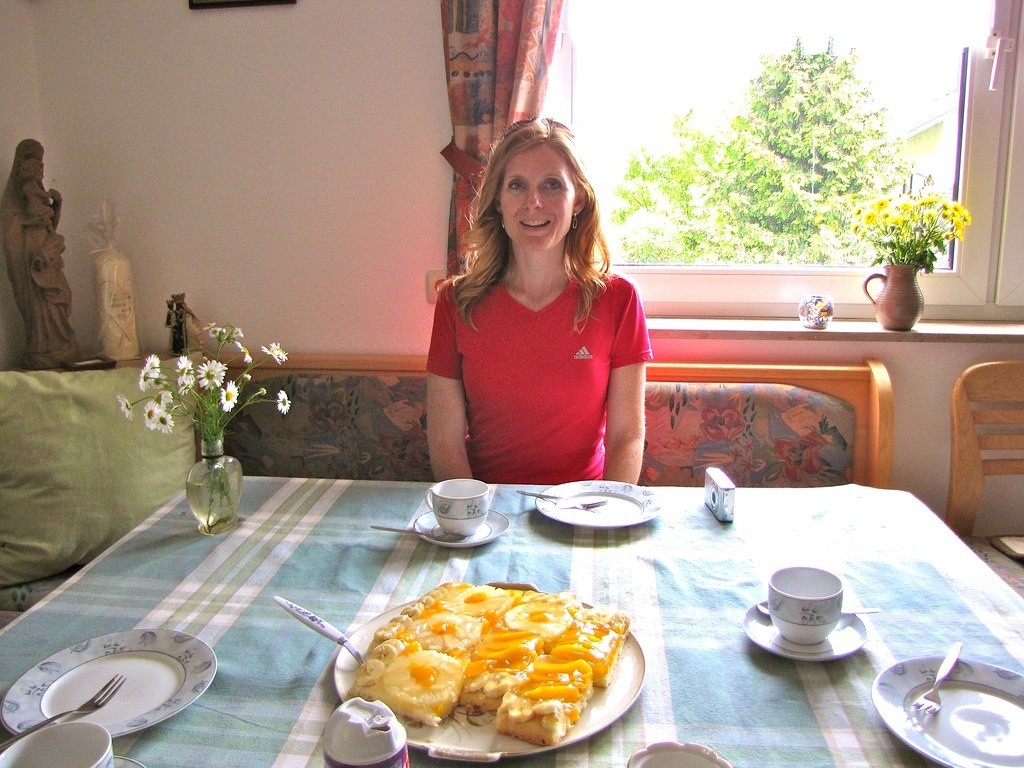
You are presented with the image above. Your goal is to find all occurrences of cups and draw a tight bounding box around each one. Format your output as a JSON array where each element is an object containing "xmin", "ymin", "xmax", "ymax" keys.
[
  {"xmin": 425, "ymin": 479, "xmax": 489, "ymax": 536},
  {"xmin": 627, "ymin": 741, "xmax": 732, "ymax": 768},
  {"xmin": 0, "ymin": 722, "xmax": 115, "ymax": 768},
  {"xmin": 768, "ymin": 567, "xmax": 844, "ymax": 646}
]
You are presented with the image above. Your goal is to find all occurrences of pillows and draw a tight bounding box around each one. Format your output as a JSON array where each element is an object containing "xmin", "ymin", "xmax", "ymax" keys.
[{"xmin": 0, "ymin": 357, "xmax": 199, "ymax": 583}]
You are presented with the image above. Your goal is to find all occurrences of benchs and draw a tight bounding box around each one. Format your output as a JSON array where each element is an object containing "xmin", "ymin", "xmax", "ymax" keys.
[{"xmin": 0, "ymin": 352, "xmax": 901, "ymax": 630}]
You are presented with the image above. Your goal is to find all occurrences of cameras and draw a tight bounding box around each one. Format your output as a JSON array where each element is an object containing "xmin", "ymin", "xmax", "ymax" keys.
[{"xmin": 705, "ymin": 467, "xmax": 736, "ymax": 523}]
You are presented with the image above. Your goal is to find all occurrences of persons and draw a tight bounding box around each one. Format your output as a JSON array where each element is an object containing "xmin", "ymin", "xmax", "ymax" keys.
[
  {"xmin": 0, "ymin": 141, "xmax": 77, "ymax": 356},
  {"xmin": 165, "ymin": 293, "xmax": 192, "ymax": 357},
  {"xmin": 427, "ymin": 118, "xmax": 645, "ymax": 486}
]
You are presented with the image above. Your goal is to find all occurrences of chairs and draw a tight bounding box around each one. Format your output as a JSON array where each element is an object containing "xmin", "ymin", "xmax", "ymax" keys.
[{"xmin": 945, "ymin": 359, "xmax": 1024, "ymax": 598}]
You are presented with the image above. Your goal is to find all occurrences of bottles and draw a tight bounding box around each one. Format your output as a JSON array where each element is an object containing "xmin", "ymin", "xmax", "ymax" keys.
[
  {"xmin": 323, "ymin": 697, "xmax": 410, "ymax": 768},
  {"xmin": 798, "ymin": 296, "xmax": 834, "ymax": 329},
  {"xmin": 96, "ymin": 250, "xmax": 139, "ymax": 360}
]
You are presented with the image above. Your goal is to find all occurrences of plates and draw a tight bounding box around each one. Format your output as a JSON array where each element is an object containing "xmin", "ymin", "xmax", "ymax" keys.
[
  {"xmin": 0, "ymin": 629, "xmax": 218, "ymax": 738},
  {"xmin": 872, "ymin": 655, "xmax": 1024, "ymax": 768},
  {"xmin": 113, "ymin": 756, "xmax": 147, "ymax": 768},
  {"xmin": 334, "ymin": 582, "xmax": 647, "ymax": 761},
  {"xmin": 742, "ymin": 600, "xmax": 869, "ymax": 661},
  {"xmin": 535, "ymin": 481, "xmax": 665, "ymax": 528},
  {"xmin": 413, "ymin": 509, "xmax": 510, "ymax": 547}
]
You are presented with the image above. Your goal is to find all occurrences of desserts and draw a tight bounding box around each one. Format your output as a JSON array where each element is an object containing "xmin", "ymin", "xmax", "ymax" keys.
[{"xmin": 350, "ymin": 580, "xmax": 631, "ymax": 748}]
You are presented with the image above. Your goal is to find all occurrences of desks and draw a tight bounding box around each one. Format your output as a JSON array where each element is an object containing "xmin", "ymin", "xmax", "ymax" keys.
[{"xmin": 0, "ymin": 475, "xmax": 1024, "ymax": 768}]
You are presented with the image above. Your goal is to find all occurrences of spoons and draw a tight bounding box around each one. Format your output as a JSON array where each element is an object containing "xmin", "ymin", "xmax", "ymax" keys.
[
  {"xmin": 370, "ymin": 525, "xmax": 468, "ymax": 542},
  {"xmin": 757, "ymin": 602, "xmax": 883, "ymax": 615}
]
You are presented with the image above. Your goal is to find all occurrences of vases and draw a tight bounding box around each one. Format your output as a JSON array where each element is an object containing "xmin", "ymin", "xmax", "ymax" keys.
[
  {"xmin": 864, "ymin": 263, "xmax": 928, "ymax": 331},
  {"xmin": 182, "ymin": 435, "xmax": 249, "ymax": 530}
]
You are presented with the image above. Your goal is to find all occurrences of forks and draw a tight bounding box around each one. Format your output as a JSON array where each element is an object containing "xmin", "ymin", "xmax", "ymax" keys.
[
  {"xmin": 516, "ymin": 490, "xmax": 607, "ymax": 510},
  {"xmin": 0, "ymin": 673, "xmax": 127, "ymax": 751},
  {"xmin": 911, "ymin": 642, "xmax": 964, "ymax": 714}
]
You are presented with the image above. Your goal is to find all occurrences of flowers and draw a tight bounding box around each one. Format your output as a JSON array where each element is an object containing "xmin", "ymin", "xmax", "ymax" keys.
[
  {"xmin": 854, "ymin": 193, "xmax": 972, "ymax": 276},
  {"xmin": 116, "ymin": 319, "xmax": 299, "ymax": 526}
]
